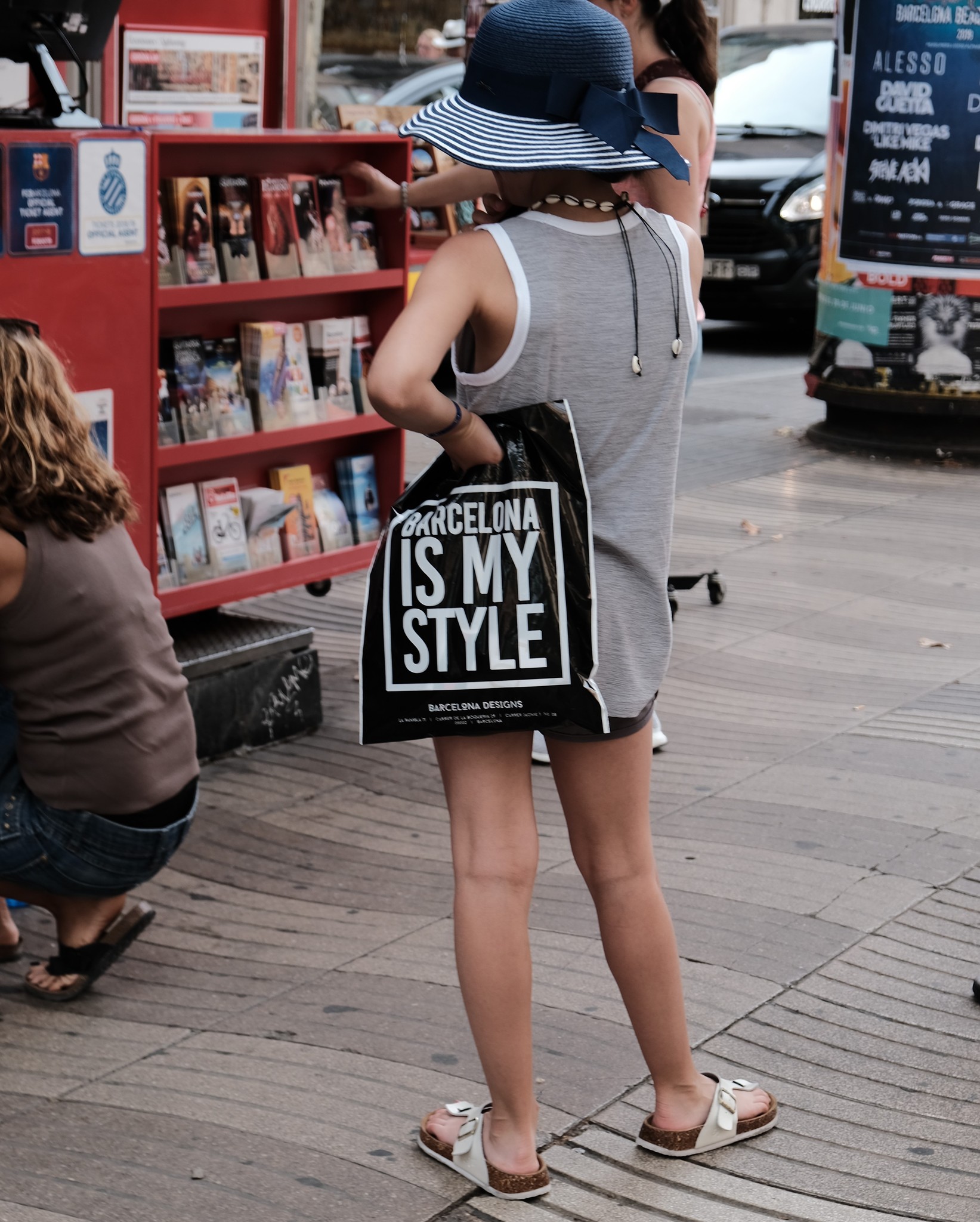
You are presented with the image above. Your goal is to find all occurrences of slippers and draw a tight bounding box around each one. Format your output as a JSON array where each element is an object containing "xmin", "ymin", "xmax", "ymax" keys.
[
  {"xmin": 420, "ymin": 1097, "xmax": 553, "ymax": 1202},
  {"xmin": 636, "ymin": 1070, "xmax": 778, "ymax": 1159},
  {"xmin": 22, "ymin": 903, "xmax": 158, "ymax": 1000}
]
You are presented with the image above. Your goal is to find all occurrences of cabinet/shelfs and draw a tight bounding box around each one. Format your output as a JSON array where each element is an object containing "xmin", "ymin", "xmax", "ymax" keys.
[{"xmin": 149, "ymin": 126, "xmax": 412, "ymax": 615}]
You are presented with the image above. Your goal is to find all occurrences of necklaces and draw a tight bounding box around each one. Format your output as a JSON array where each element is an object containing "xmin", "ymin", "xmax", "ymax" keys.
[{"xmin": 527, "ymin": 191, "xmax": 684, "ymax": 376}]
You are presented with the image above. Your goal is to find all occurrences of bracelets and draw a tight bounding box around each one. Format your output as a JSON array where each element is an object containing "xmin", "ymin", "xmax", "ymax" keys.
[
  {"xmin": 401, "ymin": 180, "xmax": 407, "ymax": 209},
  {"xmin": 427, "ymin": 399, "xmax": 461, "ymax": 438}
]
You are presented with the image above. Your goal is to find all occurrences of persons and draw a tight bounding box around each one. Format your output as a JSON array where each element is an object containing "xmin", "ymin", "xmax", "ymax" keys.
[
  {"xmin": 415, "ymin": 27, "xmax": 447, "ymax": 61},
  {"xmin": 368, "ymin": 0, "xmax": 781, "ymax": 1209},
  {"xmin": 1, "ymin": 312, "xmax": 200, "ymax": 1006},
  {"xmin": 331, "ymin": 0, "xmax": 720, "ymax": 770}
]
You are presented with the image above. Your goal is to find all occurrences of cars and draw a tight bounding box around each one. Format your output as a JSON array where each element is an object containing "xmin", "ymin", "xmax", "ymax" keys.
[
  {"xmin": 696, "ymin": 24, "xmax": 838, "ymax": 352},
  {"xmin": 311, "ymin": 54, "xmax": 469, "ymax": 174}
]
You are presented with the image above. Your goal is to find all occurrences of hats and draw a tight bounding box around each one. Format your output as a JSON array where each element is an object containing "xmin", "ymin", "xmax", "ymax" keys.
[{"xmin": 395, "ymin": 2, "xmax": 695, "ymax": 185}]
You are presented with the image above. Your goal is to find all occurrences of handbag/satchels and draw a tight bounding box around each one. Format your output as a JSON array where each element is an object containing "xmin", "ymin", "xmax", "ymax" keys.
[{"xmin": 356, "ymin": 390, "xmax": 613, "ymax": 743}]
